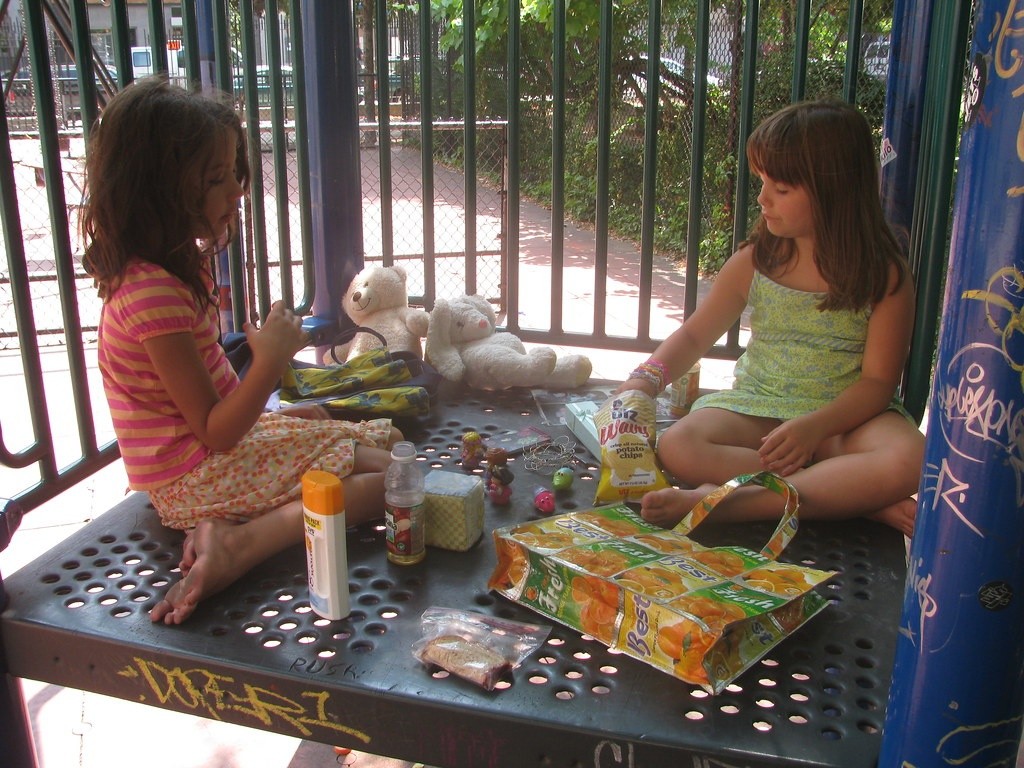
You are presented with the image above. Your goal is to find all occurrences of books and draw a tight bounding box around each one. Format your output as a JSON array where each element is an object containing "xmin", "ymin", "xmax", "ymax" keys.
[{"xmin": 480, "ymin": 425, "xmax": 551, "ymax": 457}]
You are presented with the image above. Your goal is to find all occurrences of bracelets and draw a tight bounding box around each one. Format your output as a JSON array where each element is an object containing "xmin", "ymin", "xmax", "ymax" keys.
[{"xmin": 627, "ymin": 359, "xmax": 670, "ymax": 399}]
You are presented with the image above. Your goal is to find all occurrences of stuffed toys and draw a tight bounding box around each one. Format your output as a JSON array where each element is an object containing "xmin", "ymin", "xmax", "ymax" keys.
[
  {"xmin": 426, "ymin": 294, "xmax": 592, "ymax": 388},
  {"xmin": 323, "ymin": 265, "xmax": 428, "ymax": 359}
]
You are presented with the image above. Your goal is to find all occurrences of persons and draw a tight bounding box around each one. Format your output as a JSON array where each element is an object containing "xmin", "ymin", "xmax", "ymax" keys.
[
  {"xmin": 618, "ymin": 103, "xmax": 925, "ymax": 538},
  {"xmin": 460, "ymin": 431, "xmax": 486, "ymax": 469},
  {"xmin": 78, "ymin": 73, "xmax": 407, "ymax": 624},
  {"xmin": 482, "ymin": 448, "xmax": 514, "ymax": 504}
]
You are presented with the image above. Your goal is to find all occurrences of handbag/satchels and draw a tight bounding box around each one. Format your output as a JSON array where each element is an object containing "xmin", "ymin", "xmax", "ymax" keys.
[
  {"xmin": 279, "ymin": 347, "xmax": 448, "ymax": 417},
  {"xmin": 486, "ymin": 471, "xmax": 837, "ymax": 696}
]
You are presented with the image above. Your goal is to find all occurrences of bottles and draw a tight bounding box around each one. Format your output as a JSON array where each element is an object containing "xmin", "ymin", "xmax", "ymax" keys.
[
  {"xmin": 383, "ymin": 440, "xmax": 426, "ymax": 567},
  {"xmin": 670, "ymin": 361, "xmax": 702, "ymax": 419}
]
